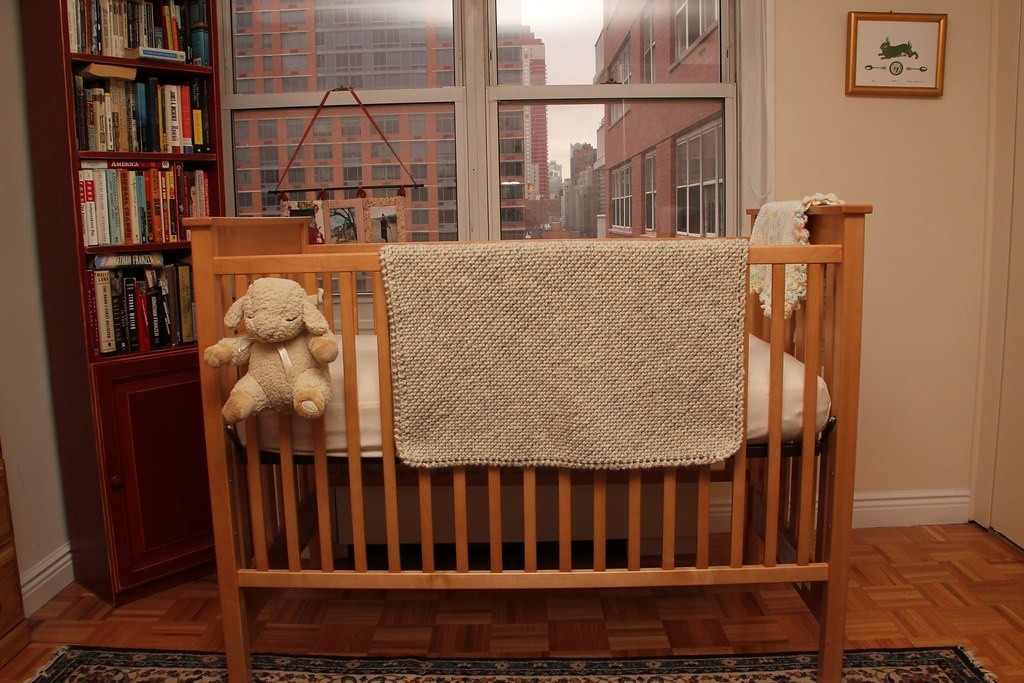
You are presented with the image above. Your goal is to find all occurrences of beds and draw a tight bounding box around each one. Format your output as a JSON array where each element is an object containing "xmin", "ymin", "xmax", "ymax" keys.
[{"xmin": 183, "ymin": 204, "xmax": 875, "ymax": 683}]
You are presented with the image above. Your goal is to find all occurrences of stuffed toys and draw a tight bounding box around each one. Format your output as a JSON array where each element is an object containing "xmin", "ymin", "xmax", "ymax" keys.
[{"xmin": 203, "ymin": 277, "xmax": 339, "ymax": 427}]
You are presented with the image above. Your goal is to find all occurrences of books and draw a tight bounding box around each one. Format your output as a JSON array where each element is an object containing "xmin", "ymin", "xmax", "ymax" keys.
[{"xmin": 67, "ymin": 0, "xmax": 209, "ymax": 354}]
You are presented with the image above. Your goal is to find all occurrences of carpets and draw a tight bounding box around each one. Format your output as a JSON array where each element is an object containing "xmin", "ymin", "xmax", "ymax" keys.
[{"xmin": 27, "ymin": 644, "xmax": 998, "ymax": 683}]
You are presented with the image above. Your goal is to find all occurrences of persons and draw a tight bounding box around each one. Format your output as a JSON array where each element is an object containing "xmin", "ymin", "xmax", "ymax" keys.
[{"xmin": 380, "ymin": 213, "xmax": 392, "ymax": 242}]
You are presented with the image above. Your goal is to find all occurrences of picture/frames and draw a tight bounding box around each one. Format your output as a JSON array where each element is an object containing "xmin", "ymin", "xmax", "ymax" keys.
[
  {"xmin": 845, "ymin": 10, "xmax": 947, "ymax": 97},
  {"xmin": 280, "ymin": 200, "xmax": 325, "ymax": 244},
  {"xmin": 363, "ymin": 196, "xmax": 407, "ymax": 244},
  {"xmin": 322, "ymin": 197, "xmax": 365, "ymax": 245}
]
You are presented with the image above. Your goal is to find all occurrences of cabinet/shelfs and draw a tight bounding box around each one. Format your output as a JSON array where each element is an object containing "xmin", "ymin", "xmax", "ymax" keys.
[{"xmin": 17, "ymin": 0, "xmax": 223, "ymax": 607}]
